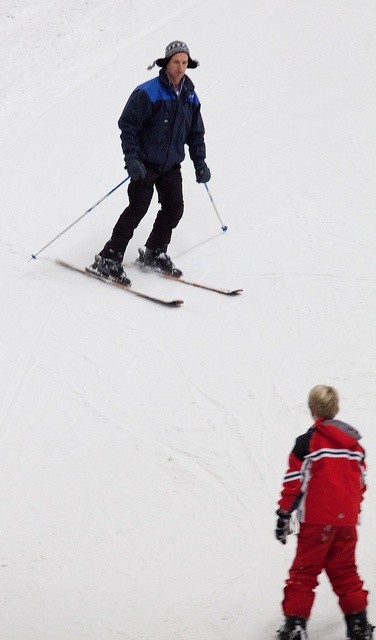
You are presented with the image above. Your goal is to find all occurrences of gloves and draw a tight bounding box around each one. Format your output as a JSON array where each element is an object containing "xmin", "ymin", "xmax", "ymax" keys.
[
  {"xmin": 124, "ymin": 154, "xmax": 146, "ymax": 181},
  {"xmin": 275, "ymin": 509, "xmax": 293, "ymax": 545},
  {"xmin": 195, "ymin": 163, "xmax": 211, "ymax": 183}
]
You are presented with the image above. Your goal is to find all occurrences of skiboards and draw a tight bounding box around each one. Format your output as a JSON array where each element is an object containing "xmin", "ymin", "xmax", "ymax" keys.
[{"xmin": 54, "ymin": 247, "xmax": 244, "ymax": 310}]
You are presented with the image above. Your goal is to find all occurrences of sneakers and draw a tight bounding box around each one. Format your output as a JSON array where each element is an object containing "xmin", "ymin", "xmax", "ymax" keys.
[
  {"xmin": 144, "ymin": 247, "xmax": 173, "ymax": 268},
  {"xmin": 276, "ymin": 615, "xmax": 307, "ymax": 640},
  {"xmin": 345, "ymin": 610, "xmax": 375, "ymax": 640},
  {"xmin": 91, "ymin": 249, "xmax": 124, "ymax": 277}
]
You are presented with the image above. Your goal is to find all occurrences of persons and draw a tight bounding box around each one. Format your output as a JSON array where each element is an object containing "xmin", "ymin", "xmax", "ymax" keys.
[
  {"xmin": 274, "ymin": 384, "xmax": 373, "ymax": 638},
  {"xmin": 87, "ymin": 40, "xmax": 211, "ymax": 288}
]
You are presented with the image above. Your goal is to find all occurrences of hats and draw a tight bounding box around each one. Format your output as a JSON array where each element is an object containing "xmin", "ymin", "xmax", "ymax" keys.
[{"xmin": 147, "ymin": 40, "xmax": 199, "ymax": 70}]
